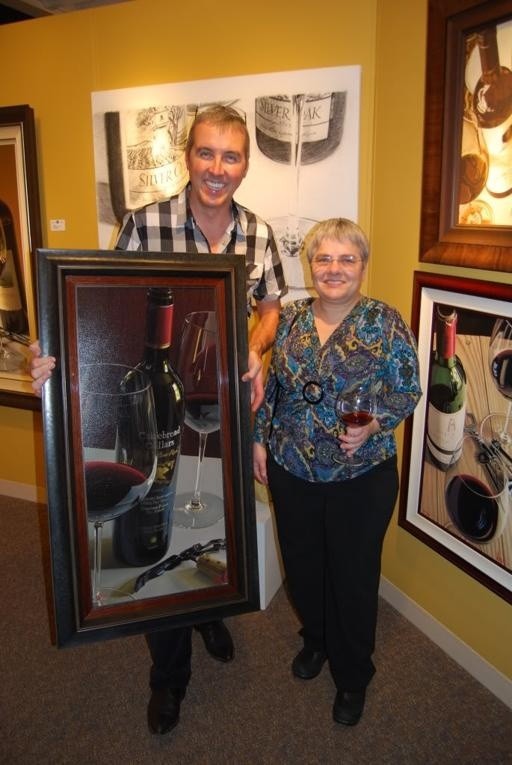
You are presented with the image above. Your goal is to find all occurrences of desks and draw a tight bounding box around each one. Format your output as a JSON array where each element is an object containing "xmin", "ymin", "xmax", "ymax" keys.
[
  {"xmin": 82, "ymin": 446, "xmax": 229, "ymax": 608},
  {"xmin": 418, "ymin": 332, "xmax": 512, "ymax": 572}
]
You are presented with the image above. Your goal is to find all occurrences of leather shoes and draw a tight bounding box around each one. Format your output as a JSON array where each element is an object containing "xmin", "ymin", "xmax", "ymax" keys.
[
  {"xmin": 147, "ymin": 688, "xmax": 186, "ymax": 734},
  {"xmin": 199, "ymin": 620, "xmax": 234, "ymax": 663}
]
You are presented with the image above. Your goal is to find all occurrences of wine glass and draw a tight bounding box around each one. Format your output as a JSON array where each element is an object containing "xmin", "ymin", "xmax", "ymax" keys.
[
  {"xmin": 261, "ymin": 94, "xmax": 328, "ymax": 290},
  {"xmin": 172, "ymin": 308, "xmax": 226, "ymax": 531},
  {"xmin": 334, "ymin": 389, "xmax": 377, "ymax": 468},
  {"xmin": 478, "ymin": 314, "xmax": 511, "ymax": 465},
  {"xmin": 80, "ymin": 363, "xmax": 156, "ymax": 608},
  {"xmin": 458, "ymin": 92, "xmax": 491, "ymax": 228},
  {"xmin": 446, "ymin": 437, "xmax": 508, "ymax": 546}
]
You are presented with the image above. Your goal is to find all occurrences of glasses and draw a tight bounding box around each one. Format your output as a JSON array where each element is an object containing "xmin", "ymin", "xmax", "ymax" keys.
[{"xmin": 315, "ymin": 256, "xmax": 363, "ymax": 268}]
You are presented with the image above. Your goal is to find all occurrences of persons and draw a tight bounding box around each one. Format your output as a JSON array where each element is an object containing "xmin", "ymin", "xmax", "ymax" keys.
[
  {"xmin": 250, "ymin": 217, "xmax": 424, "ymax": 727},
  {"xmin": 27, "ymin": 107, "xmax": 289, "ymax": 736}
]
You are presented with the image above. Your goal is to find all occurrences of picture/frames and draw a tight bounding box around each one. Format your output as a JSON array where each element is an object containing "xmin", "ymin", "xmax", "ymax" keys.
[
  {"xmin": 33, "ymin": 245, "xmax": 261, "ymax": 647},
  {"xmin": 398, "ymin": 270, "xmax": 512, "ymax": 603},
  {"xmin": 417, "ymin": 1, "xmax": 512, "ymax": 273},
  {"xmin": 0, "ymin": 104, "xmax": 44, "ymax": 412}
]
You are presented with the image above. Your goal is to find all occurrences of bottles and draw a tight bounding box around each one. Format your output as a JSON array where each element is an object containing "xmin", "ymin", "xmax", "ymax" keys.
[
  {"xmin": 113, "ymin": 289, "xmax": 185, "ymax": 569},
  {"xmin": 2, "ymin": 196, "xmax": 29, "ymax": 335},
  {"xmin": 251, "ymin": 93, "xmax": 347, "ymax": 168},
  {"xmin": 475, "ymin": 27, "xmax": 511, "ymax": 199},
  {"xmin": 425, "ymin": 305, "xmax": 468, "ymax": 470},
  {"xmin": 102, "ymin": 108, "xmax": 191, "ymax": 222}
]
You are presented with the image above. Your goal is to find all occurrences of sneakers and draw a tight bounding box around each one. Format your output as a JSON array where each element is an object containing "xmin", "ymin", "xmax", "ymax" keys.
[
  {"xmin": 293, "ymin": 647, "xmax": 327, "ymax": 679},
  {"xmin": 333, "ymin": 686, "xmax": 366, "ymax": 726}
]
